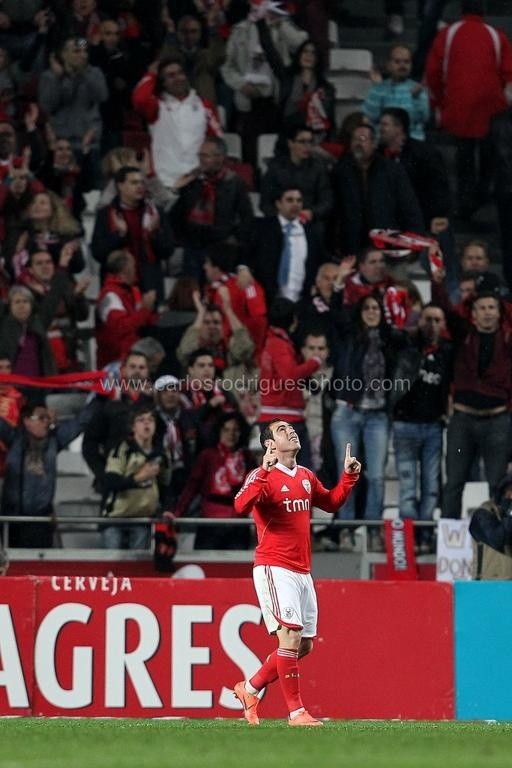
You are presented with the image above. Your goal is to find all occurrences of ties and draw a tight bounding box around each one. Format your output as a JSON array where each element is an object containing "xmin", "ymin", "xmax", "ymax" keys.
[{"xmin": 276, "ymin": 224, "xmax": 295, "ymax": 287}]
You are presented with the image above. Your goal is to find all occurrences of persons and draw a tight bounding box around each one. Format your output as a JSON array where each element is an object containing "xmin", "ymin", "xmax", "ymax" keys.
[
  {"xmin": 0, "ymin": 0, "xmax": 511, "ymax": 580},
  {"xmin": 230, "ymin": 419, "xmax": 362, "ymax": 726}
]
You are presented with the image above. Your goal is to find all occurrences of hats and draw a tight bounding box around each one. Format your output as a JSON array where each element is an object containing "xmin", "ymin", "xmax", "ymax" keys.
[{"xmin": 154, "ymin": 375, "xmax": 178, "ymax": 389}]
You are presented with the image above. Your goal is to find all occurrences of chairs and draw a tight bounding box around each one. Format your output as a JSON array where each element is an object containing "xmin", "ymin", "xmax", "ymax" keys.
[{"xmin": 52, "ymin": 17, "xmax": 492, "ymax": 551}]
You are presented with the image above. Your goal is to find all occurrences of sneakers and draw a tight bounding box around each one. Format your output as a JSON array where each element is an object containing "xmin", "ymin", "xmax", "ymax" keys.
[
  {"xmin": 288, "ymin": 712, "xmax": 324, "ymax": 726},
  {"xmin": 233, "ymin": 680, "xmax": 259, "ymax": 725}
]
